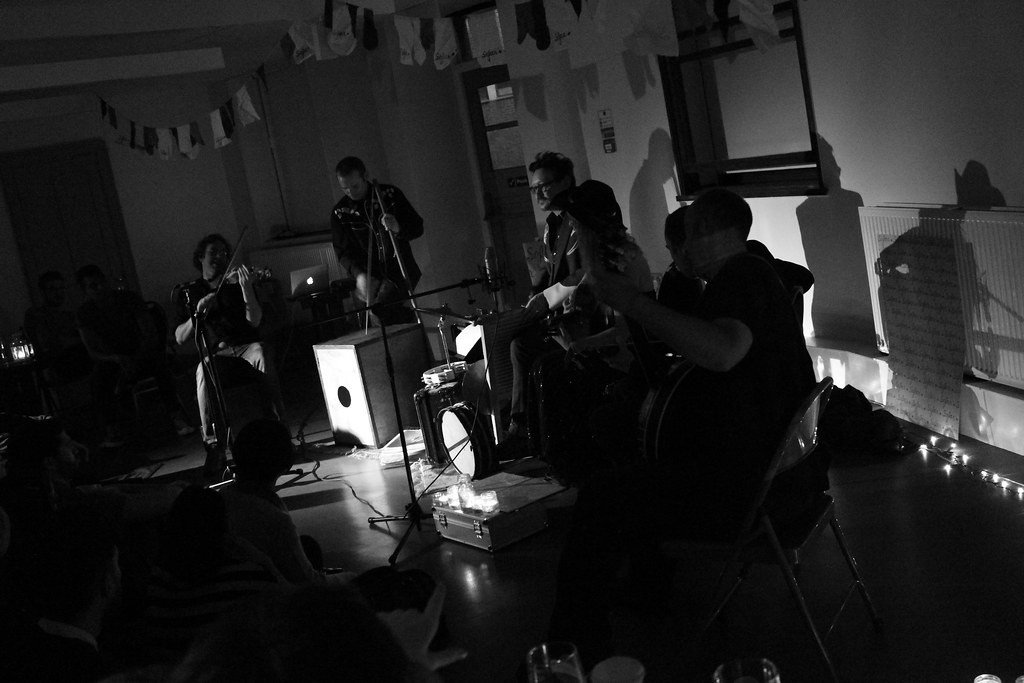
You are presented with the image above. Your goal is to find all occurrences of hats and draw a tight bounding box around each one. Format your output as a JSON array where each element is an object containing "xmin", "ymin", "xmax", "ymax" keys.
[{"xmin": 545, "ymin": 180, "xmax": 628, "ymax": 234}]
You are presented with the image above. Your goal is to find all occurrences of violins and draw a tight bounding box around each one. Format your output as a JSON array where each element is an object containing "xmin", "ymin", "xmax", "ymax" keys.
[{"xmin": 223, "ymin": 266, "xmax": 263, "ymax": 284}]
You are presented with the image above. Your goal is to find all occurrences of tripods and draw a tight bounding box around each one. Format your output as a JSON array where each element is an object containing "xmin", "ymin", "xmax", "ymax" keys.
[
  {"xmin": 363, "ymin": 277, "xmax": 488, "ymax": 574},
  {"xmin": 189, "ymin": 303, "xmax": 235, "ymax": 481}
]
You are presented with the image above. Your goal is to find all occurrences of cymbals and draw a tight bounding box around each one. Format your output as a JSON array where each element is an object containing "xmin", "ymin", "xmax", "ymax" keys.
[
  {"xmin": 410, "ymin": 305, "xmax": 478, "ymax": 329},
  {"xmin": 421, "ymin": 361, "xmax": 469, "ymax": 385}
]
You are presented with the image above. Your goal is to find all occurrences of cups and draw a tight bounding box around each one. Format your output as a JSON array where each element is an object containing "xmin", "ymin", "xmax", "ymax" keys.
[
  {"xmin": 592, "ymin": 658, "xmax": 643, "ymax": 682},
  {"xmin": 526, "ymin": 641, "xmax": 583, "ymax": 681},
  {"xmin": 434, "ymin": 472, "xmax": 501, "ymax": 516},
  {"xmin": 712, "ymin": 658, "xmax": 780, "ymax": 682}
]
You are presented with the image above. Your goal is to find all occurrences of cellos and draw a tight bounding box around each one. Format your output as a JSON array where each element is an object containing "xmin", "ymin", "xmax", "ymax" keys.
[{"xmin": 542, "ymin": 184, "xmax": 723, "ymax": 509}]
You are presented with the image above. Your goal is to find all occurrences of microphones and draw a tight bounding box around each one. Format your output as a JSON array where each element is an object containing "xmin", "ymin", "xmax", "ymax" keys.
[
  {"xmin": 176, "ymin": 279, "xmax": 197, "ymax": 290},
  {"xmin": 483, "ymin": 245, "xmax": 498, "ymax": 300},
  {"xmin": 192, "ymin": 312, "xmax": 203, "ymax": 342}
]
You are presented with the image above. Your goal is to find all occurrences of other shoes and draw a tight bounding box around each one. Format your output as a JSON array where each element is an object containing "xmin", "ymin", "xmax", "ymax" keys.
[
  {"xmin": 97, "ymin": 425, "xmax": 124, "ymax": 448},
  {"xmin": 169, "ymin": 412, "xmax": 195, "ymax": 436},
  {"xmin": 202, "ymin": 443, "xmax": 226, "ymax": 482}
]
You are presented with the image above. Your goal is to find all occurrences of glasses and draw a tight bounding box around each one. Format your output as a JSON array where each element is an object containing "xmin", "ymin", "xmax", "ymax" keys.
[{"xmin": 528, "ymin": 178, "xmax": 555, "ymax": 195}]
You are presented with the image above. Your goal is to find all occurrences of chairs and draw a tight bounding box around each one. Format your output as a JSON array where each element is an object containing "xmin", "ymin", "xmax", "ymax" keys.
[{"xmin": 678, "ymin": 377, "xmax": 882, "ymax": 683}]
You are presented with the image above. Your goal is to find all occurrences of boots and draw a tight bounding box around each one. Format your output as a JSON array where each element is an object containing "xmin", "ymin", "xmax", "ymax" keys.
[{"xmin": 495, "ymin": 411, "xmax": 538, "ymax": 460}]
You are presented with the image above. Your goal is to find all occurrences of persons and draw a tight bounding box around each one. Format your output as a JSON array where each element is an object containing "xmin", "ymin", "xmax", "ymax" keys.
[
  {"xmin": 676, "ymin": 187, "xmax": 817, "ymax": 484},
  {"xmin": 656, "ymin": 209, "xmax": 716, "ymax": 323},
  {"xmin": 325, "ymin": 150, "xmax": 426, "ymax": 337},
  {"xmin": 557, "ymin": 178, "xmax": 658, "ymax": 385},
  {"xmin": 165, "ymin": 232, "xmax": 301, "ymax": 496},
  {"xmin": 510, "ymin": 146, "xmax": 593, "ymax": 443}
]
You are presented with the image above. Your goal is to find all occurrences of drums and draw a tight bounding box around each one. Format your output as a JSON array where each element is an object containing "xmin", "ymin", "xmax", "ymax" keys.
[{"xmin": 433, "ymin": 401, "xmax": 500, "ymax": 480}]
